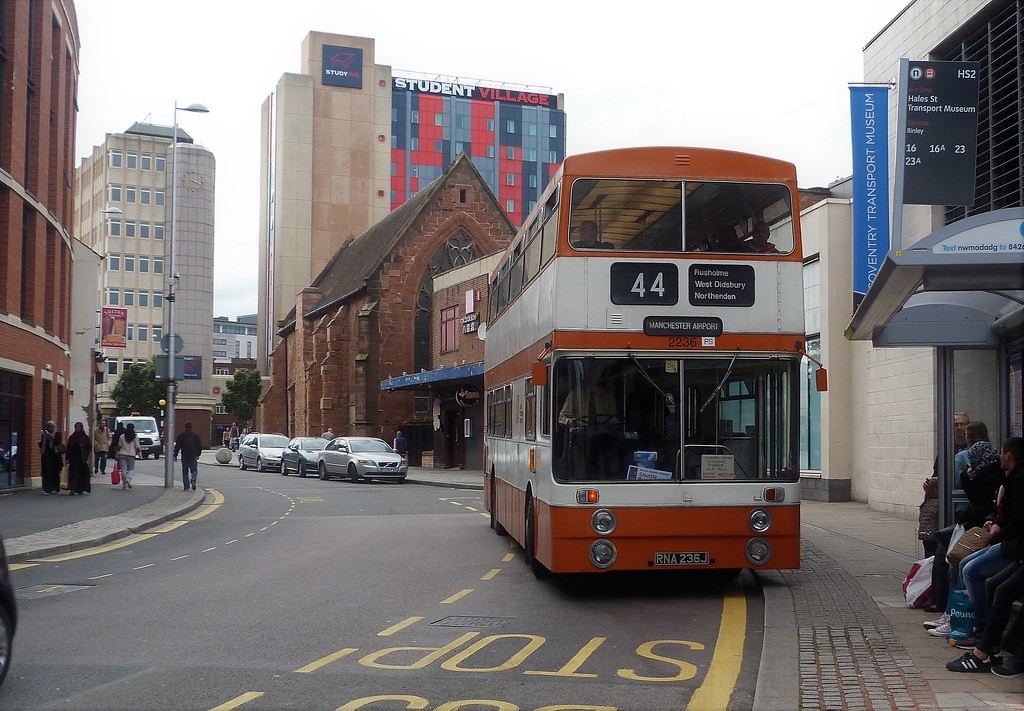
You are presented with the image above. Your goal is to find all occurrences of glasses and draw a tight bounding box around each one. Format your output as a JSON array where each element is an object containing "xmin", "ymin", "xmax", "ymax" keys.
[{"xmin": 47, "ymin": 426, "xmax": 53, "ymax": 429}]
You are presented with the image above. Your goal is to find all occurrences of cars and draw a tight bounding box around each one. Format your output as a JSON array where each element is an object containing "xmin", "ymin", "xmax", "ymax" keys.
[
  {"xmin": 281, "ymin": 437, "xmax": 334, "ymax": 477},
  {"xmin": 238, "ymin": 431, "xmax": 292, "ymax": 472},
  {"xmin": 318, "ymin": 436, "xmax": 408, "ymax": 484}
]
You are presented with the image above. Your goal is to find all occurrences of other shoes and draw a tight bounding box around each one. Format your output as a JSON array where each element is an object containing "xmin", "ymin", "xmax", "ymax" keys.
[
  {"xmin": 126, "ymin": 481, "xmax": 132, "ymax": 489},
  {"xmin": 190, "ymin": 481, "xmax": 196, "ymax": 490},
  {"xmin": 924, "ymin": 605, "xmax": 946, "ymax": 613},
  {"xmin": 123, "ymin": 487, "xmax": 127, "ymax": 490}
]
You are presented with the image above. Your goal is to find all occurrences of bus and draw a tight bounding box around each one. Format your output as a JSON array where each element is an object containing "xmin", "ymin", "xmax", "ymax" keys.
[{"xmin": 481, "ymin": 147, "xmax": 828, "ymax": 579}]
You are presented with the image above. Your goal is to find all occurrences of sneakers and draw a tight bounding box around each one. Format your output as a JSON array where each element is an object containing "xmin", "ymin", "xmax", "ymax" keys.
[
  {"xmin": 973, "ymin": 632, "xmax": 984, "ymax": 640},
  {"xmin": 992, "ymin": 652, "xmax": 1004, "ymax": 666},
  {"xmin": 955, "ymin": 638, "xmax": 1000, "ymax": 654},
  {"xmin": 923, "ymin": 613, "xmax": 950, "ymax": 630},
  {"xmin": 927, "ymin": 621, "xmax": 950, "ymax": 637},
  {"xmin": 945, "ymin": 650, "xmax": 991, "ymax": 672},
  {"xmin": 991, "ymin": 657, "xmax": 1024, "ymax": 678}
]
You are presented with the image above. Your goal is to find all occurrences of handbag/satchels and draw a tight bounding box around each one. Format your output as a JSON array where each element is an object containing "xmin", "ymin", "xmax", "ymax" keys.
[
  {"xmin": 949, "ymin": 589, "xmax": 974, "ymax": 638},
  {"xmin": 52, "ymin": 441, "xmax": 68, "ymax": 455},
  {"xmin": 111, "ymin": 460, "xmax": 120, "ymax": 485},
  {"xmin": 901, "ymin": 555, "xmax": 937, "ymax": 610},
  {"xmin": 947, "ymin": 522, "xmax": 992, "ymax": 569},
  {"xmin": 944, "ymin": 523, "xmax": 967, "ymax": 566}
]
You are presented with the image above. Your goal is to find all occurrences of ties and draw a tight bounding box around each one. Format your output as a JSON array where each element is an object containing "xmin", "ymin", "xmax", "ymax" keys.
[{"xmin": 588, "ymin": 389, "xmax": 597, "ymax": 423}]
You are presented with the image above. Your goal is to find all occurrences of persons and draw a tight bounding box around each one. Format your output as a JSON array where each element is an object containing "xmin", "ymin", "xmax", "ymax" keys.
[
  {"xmin": 222, "ymin": 421, "xmax": 245, "ymax": 452},
  {"xmin": 93, "ymin": 419, "xmax": 112, "ymax": 474},
  {"xmin": 173, "ymin": 422, "xmax": 201, "ymax": 491},
  {"xmin": 65, "ymin": 421, "xmax": 91, "ymax": 495},
  {"xmin": 322, "ymin": 428, "xmax": 335, "ymax": 441},
  {"xmin": 705, "ymin": 220, "xmax": 779, "ymax": 253},
  {"xmin": 38, "ymin": 421, "xmax": 62, "ymax": 495},
  {"xmin": 559, "ymin": 363, "xmax": 619, "ymax": 480},
  {"xmin": 922, "ymin": 411, "xmax": 1024, "ymax": 678},
  {"xmin": 117, "ymin": 423, "xmax": 142, "ymax": 490},
  {"xmin": 113, "ymin": 422, "xmax": 126, "ymax": 472},
  {"xmin": 394, "ymin": 431, "xmax": 408, "ymax": 459},
  {"xmin": 571, "ymin": 220, "xmax": 616, "ymax": 249}
]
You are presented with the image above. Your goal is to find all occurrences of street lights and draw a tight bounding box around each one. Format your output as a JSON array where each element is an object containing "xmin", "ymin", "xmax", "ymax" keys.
[
  {"xmin": 166, "ymin": 104, "xmax": 211, "ymax": 488},
  {"xmin": 158, "ymin": 399, "xmax": 165, "ymax": 442}
]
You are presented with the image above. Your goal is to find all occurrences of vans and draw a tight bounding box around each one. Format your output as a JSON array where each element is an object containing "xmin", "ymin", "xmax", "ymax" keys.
[{"xmin": 115, "ymin": 415, "xmax": 164, "ymax": 459}]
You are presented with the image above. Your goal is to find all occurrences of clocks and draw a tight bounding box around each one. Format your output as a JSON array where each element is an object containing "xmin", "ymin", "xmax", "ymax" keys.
[{"xmin": 181, "ymin": 170, "xmax": 205, "ymax": 192}]
